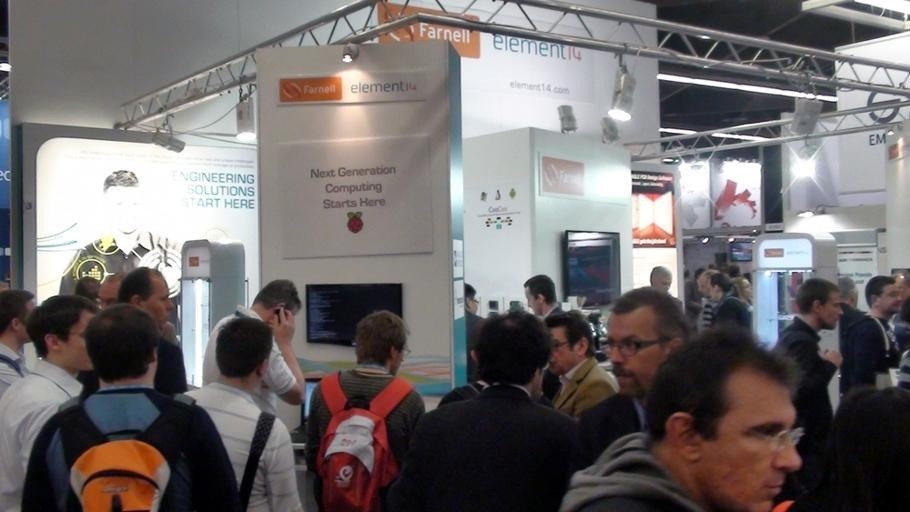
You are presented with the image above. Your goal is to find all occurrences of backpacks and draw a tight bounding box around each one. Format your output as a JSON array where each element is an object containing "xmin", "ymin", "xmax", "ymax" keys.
[
  {"xmin": 61, "ymin": 392, "xmax": 194, "ymax": 512},
  {"xmin": 315, "ymin": 372, "xmax": 414, "ymax": 511}
]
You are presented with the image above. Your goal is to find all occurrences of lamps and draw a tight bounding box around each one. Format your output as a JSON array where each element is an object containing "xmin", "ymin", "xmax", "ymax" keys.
[
  {"xmin": 555, "ymin": 103, "xmax": 621, "ymax": 143},
  {"xmin": 600, "ymin": 54, "xmax": 825, "ymax": 137},
  {"xmin": 148, "ymin": 37, "xmax": 363, "ymax": 155}
]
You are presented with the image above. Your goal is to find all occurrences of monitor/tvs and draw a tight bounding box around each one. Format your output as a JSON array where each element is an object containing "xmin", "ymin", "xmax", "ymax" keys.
[
  {"xmin": 730, "ymin": 242, "xmax": 752, "ymax": 261},
  {"xmin": 565, "ymin": 230, "xmax": 620, "ymax": 308},
  {"xmin": 304, "ymin": 283, "xmax": 403, "ymax": 348}
]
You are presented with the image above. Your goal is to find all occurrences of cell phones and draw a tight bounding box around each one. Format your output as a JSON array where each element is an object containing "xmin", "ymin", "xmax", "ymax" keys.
[{"xmin": 274, "ymin": 308, "xmax": 280, "ymax": 316}]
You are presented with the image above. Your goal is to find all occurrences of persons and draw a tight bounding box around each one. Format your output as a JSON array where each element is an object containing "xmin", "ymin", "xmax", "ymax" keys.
[
  {"xmin": 1, "ymin": 267, "xmax": 304, "ymax": 511},
  {"xmin": 772, "ymin": 275, "xmax": 910, "ymax": 512},
  {"xmin": 388, "ymin": 264, "xmax": 802, "ymax": 511},
  {"xmin": 304, "ymin": 311, "xmax": 425, "ymax": 510},
  {"xmin": 45, "ymin": 166, "xmax": 186, "ymax": 318}
]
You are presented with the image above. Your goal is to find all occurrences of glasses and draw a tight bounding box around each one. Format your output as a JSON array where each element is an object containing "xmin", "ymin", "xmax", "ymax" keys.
[
  {"xmin": 604, "ymin": 339, "xmax": 660, "ymax": 357},
  {"xmin": 736, "ymin": 424, "xmax": 804, "ymax": 451}
]
